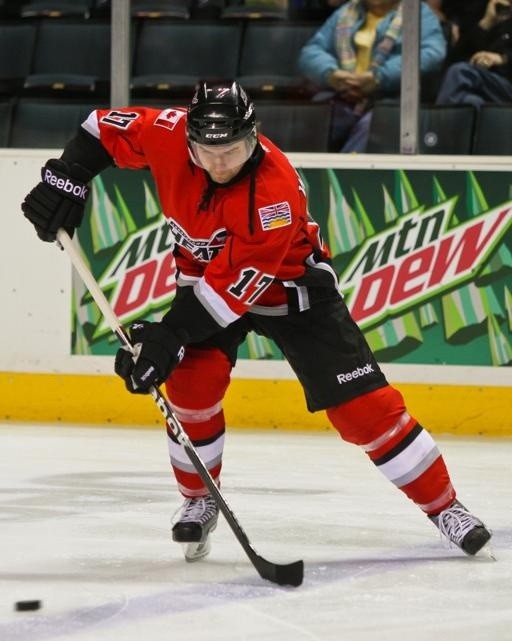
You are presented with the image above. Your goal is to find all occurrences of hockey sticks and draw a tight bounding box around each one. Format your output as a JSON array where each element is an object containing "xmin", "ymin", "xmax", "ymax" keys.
[{"xmin": 56, "ymin": 228, "xmax": 303, "ymax": 586}]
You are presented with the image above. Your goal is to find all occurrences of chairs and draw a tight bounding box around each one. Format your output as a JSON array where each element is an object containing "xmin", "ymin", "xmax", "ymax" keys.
[{"xmin": 0, "ymin": 0, "xmax": 512, "ymax": 154}]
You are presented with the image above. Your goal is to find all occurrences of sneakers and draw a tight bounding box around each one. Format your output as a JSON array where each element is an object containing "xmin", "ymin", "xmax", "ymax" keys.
[
  {"xmin": 426, "ymin": 498, "xmax": 491, "ymax": 556},
  {"xmin": 171, "ymin": 481, "xmax": 221, "ymax": 543}
]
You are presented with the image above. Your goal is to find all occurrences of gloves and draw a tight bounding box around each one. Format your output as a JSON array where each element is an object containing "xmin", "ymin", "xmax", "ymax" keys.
[
  {"xmin": 114, "ymin": 316, "xmax": 192, "ymax": 394},
  {"xmin": 21, "ymin": 158, "xmax": 94, "ymax": 251}
]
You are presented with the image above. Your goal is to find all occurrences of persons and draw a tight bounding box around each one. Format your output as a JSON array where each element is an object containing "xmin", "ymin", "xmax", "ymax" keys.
[
  {"xmin": 289, "ymin": 0, "xmax": 349, "ymax": 26},
  {"xmin": 432, "ymin": 0, "xmax": 512, "ymax": 107},
  {"xmin": 296, "ymin": 0, "xmax": 448, "ymax": 153},
  {"xmin": 22, "ymin": 78, "xmax": 492, "ymax": 555},
  {"xmin": 424, "ymin": 0, "xmax": 462, "ymax": 57}
]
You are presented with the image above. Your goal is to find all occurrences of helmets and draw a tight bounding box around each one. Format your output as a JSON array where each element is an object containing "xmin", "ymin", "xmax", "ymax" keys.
[{"xmin": 184, "ymin": 80, "xmax": 258, "ymax": 172}]
